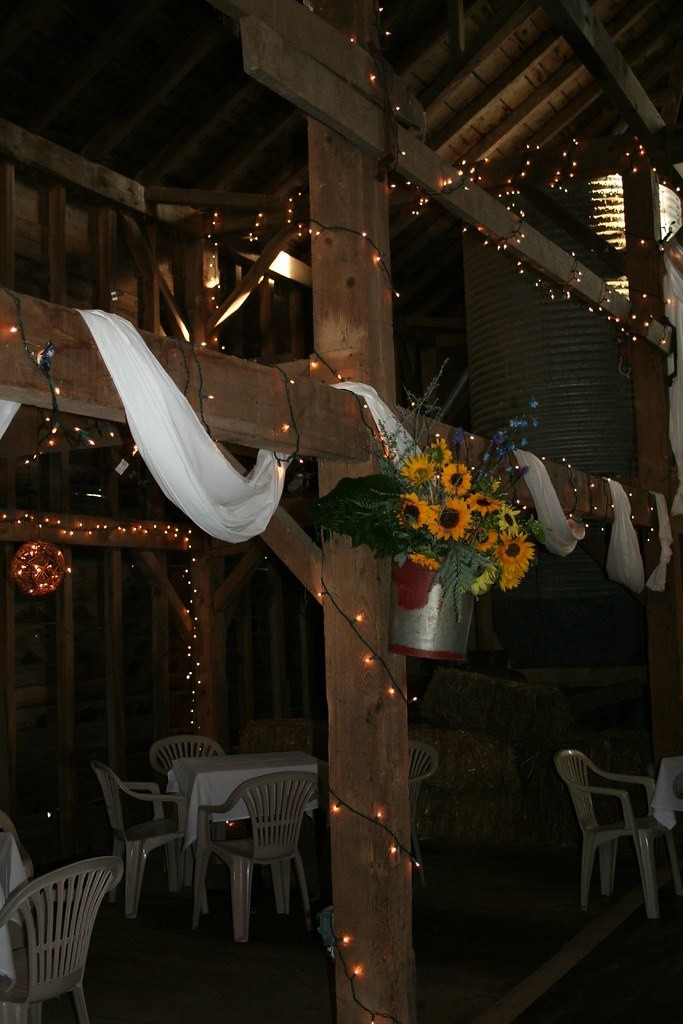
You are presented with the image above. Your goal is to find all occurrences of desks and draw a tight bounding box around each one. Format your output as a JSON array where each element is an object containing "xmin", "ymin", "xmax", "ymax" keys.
[{"xmin": 165, "ymin": 750, "xmax": 329, "ymax": 916}]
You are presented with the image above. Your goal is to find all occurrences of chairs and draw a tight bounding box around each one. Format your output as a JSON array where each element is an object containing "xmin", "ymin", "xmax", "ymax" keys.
[
  {"xmin": 0, "ymin": 735, "xmax": 440, "ymax": 1024},
  {"xmin": 553, "ymin": 749, "xmax": 683, "ymax": 920}
]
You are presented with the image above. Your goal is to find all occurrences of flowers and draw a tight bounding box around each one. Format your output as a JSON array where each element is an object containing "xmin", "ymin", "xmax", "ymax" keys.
[{"xmin": 301, "ymin": 354, "xmax": 553, "ymax": 624}]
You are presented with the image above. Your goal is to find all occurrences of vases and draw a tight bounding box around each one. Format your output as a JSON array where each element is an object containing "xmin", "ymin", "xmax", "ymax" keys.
[{"xmin": 385, "ymin": 553, "xmax": 474, "ymax": 664}]
